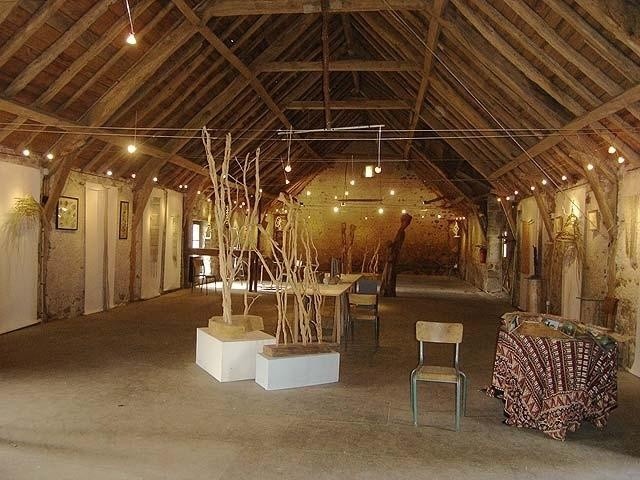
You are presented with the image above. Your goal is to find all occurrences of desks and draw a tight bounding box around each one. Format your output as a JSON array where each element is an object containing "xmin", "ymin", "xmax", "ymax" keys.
[{"xmin": 497, "ymin": 312, "xmax": 619, "ymax": 441}]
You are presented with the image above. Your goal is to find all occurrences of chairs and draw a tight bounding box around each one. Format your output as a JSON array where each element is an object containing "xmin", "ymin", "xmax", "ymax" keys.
[
  {"xmin": 408, "ymin": 319, "xmax": 468, "ymax": 433},
  {"xmin": 322, "ymin": 269, "xmax": 385, "ymax": 351}
]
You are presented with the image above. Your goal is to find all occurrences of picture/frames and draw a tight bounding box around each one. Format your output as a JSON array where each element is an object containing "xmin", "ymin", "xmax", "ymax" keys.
[
  {"xmin": 119, "ymin": 201, "xmax": 130, "ymax": 240},
  {"xmin": 55, "ymin": 194, "xmax": 79, "ymax": 231}
]
